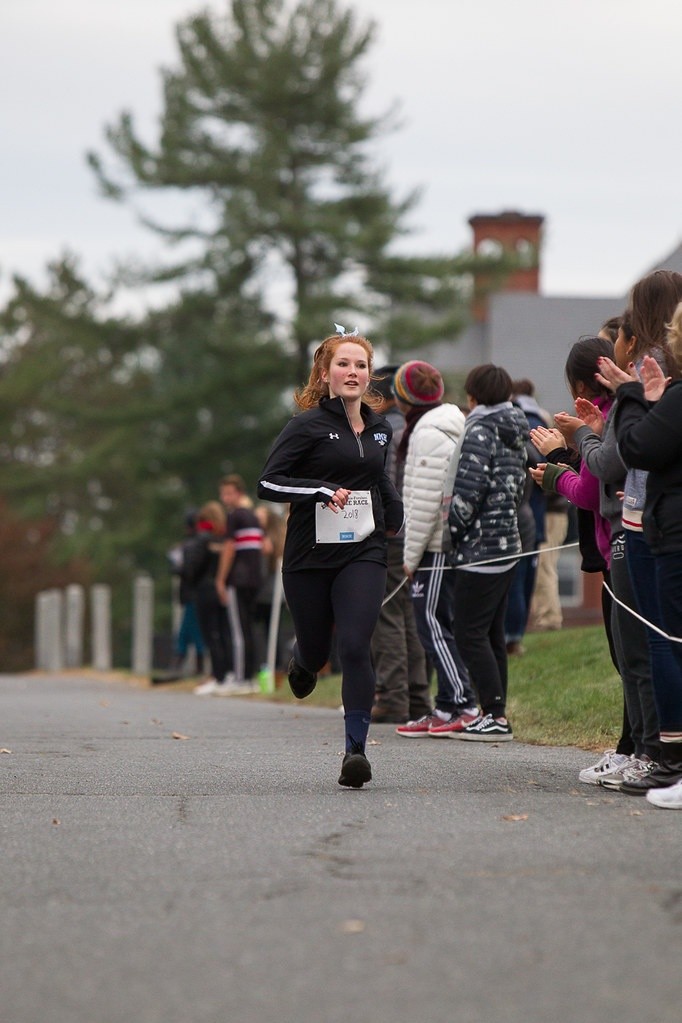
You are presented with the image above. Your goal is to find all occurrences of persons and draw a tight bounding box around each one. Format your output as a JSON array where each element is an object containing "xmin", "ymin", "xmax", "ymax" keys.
[
  {"xmin": 169, "ymin": 472, "xmax": 342, "ymax": 698},
  {"xmin": 360, "ymin": 361, "xmax": 569, "ymax": 738},
  {"xmin": 529, "ymin": 271, "xmax": 682, "ymax": 796},
  {"xmin": 255, "ymin": 323, "xmax": 405, "ymax": 789},
  {"xmin": 448, "ymin": 362, "xmax": 530, "ymax": 743},
  {"xmin": 594, "ymin": 303, "xmax": 682, "ymax": 810}
]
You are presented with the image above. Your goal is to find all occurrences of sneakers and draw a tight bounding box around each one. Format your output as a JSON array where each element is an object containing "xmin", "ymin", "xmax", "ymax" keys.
[
  {"xmin": 429, "ymin": 712, "xmax": 483, "ymax": 739},
  {"xmin": 399, "ymin": 711, "xmax": 451, "ymax": 738},
  {"xmin": 647, "ymin": 777, "xmax": 682, "ymax": 809},
  {"xmin": 288, "ymin": 658, "xmax": 318, "ymax": 699},
  {"xmin": 451, "ymin": 717, "xmax": 514, "ymax": 740},
  {"xmin": 578, "ymin": 750, "xmax": 634, "ymax": 785},
  {"xmin": 598, "ymin": 759, "xmax": 658, "ymax": 792},
  {"xmin": 338, "ymin": 751, "xmax": 371, "ymax": 788},
  {"xmin": 620, "ymin": 766, "xmax": 674, "ymax": 795}
]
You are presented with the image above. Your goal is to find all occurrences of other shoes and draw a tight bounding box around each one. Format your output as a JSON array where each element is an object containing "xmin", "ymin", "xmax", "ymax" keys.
[
  {"xmin": 370, "ymin": 704, "xmax": 408, "ymax": 724},
  {"xmin": 191, "ymin": 676, "xmax": 262, "ymax": 696},
  {"xmin": 408, "ymin": 709, "xmax": 431, "ymax": 721}
]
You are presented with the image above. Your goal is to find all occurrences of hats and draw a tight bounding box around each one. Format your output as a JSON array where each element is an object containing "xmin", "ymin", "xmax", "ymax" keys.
[
  {"xmin": 367, "ymin": 366, "xmax": 397, "ymax": 399},
  {"xmin": 392, "ymin": 361, "xmax": 444, "ymax": 405}
]
